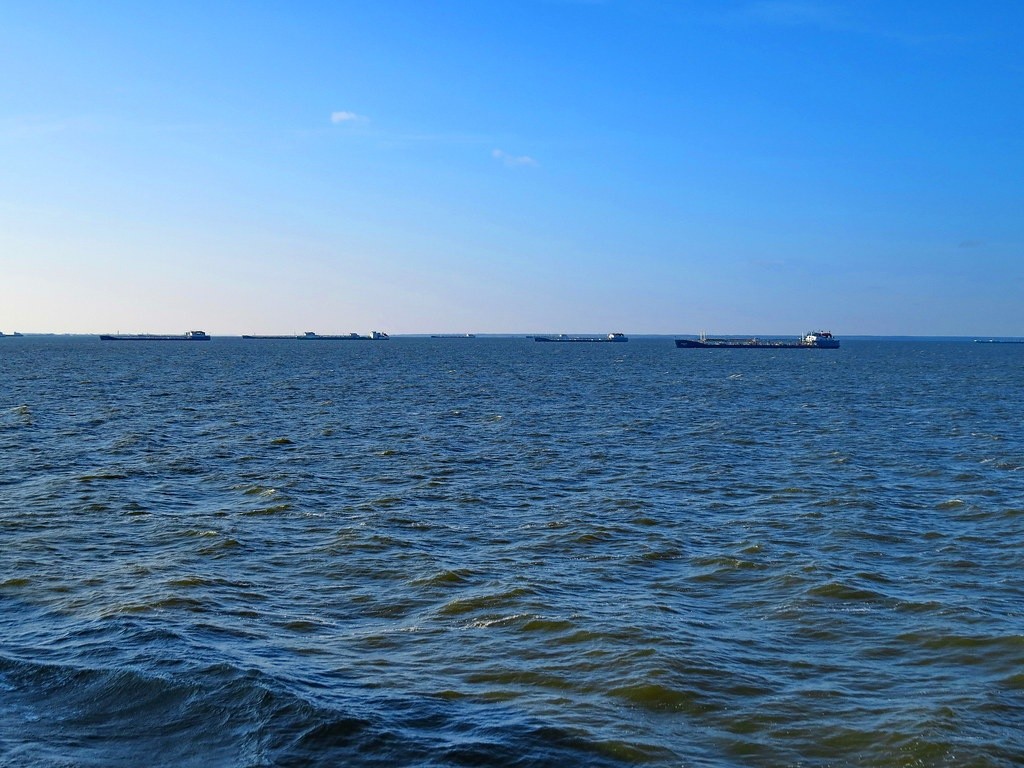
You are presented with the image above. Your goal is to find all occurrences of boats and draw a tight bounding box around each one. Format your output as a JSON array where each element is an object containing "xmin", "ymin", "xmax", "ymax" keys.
[
  {"xmin": 526, "ymin": 331, "xmax": 629, "ymax": 343},
  {"xmin": 674, "ymin": 327, "xmax": 841, "ymax": 348},
  {"xmin": 242, "ymin": 329, "xmax": 389, "ymax": 340},
  {"xmin": 98, "ymin": 329, "xmax": 211, "ymax": 340}
]
